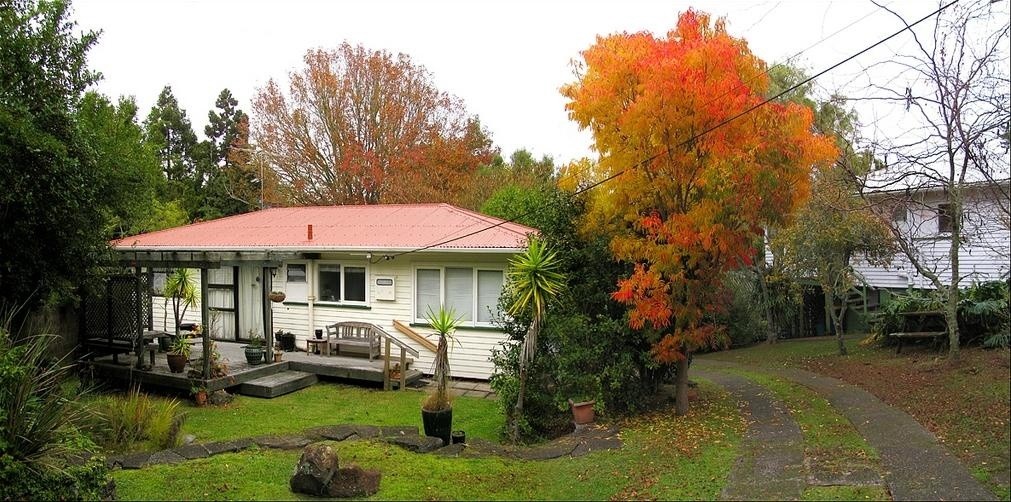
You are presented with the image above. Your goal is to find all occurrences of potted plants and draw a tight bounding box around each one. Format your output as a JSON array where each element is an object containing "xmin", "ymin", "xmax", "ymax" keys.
[
  {"xmin": 240, "ymin": 327, "xmax": 295, "ymax": 365},
  {"xmin": 418, "ymin": 301, "xmax": 469, "ymax": 446},
  {"xmin": 568, "ymin": 390, "xmax": 598, "ymax": 425},
  {"xmin": 267, "ymin": 291, "xmax": 287, "ymax": 303},
  {"xmin": 162, "ymin": 266, "xmax": 203, "ymax": 373},
  {"xmin": 189, "ymin": 386, "xmax": 208, "ymax": 407}
]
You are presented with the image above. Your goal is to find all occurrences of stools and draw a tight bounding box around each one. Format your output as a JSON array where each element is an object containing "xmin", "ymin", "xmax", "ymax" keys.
[{"xmin": 306, "ymin": 338, "xmax": 326, "ymax": 356}]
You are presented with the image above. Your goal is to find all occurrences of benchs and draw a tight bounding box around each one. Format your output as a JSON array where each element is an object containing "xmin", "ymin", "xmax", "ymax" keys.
[
  {"xmin": 888, "ymin": 310, "xmax": 949, "ymax": 354},
  {"xmin": 88, "ymin": 329, "xmax": 163, "ymax": 366},
  {"xmin": 326, "ymin": 320, "xmax": 381, "ymax": 362}
]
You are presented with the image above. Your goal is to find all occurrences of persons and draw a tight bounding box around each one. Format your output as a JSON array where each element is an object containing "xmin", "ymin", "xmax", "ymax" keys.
[{"xmin": 321, "ymin": 283, "xmax": 333, "ymax": 300}]
